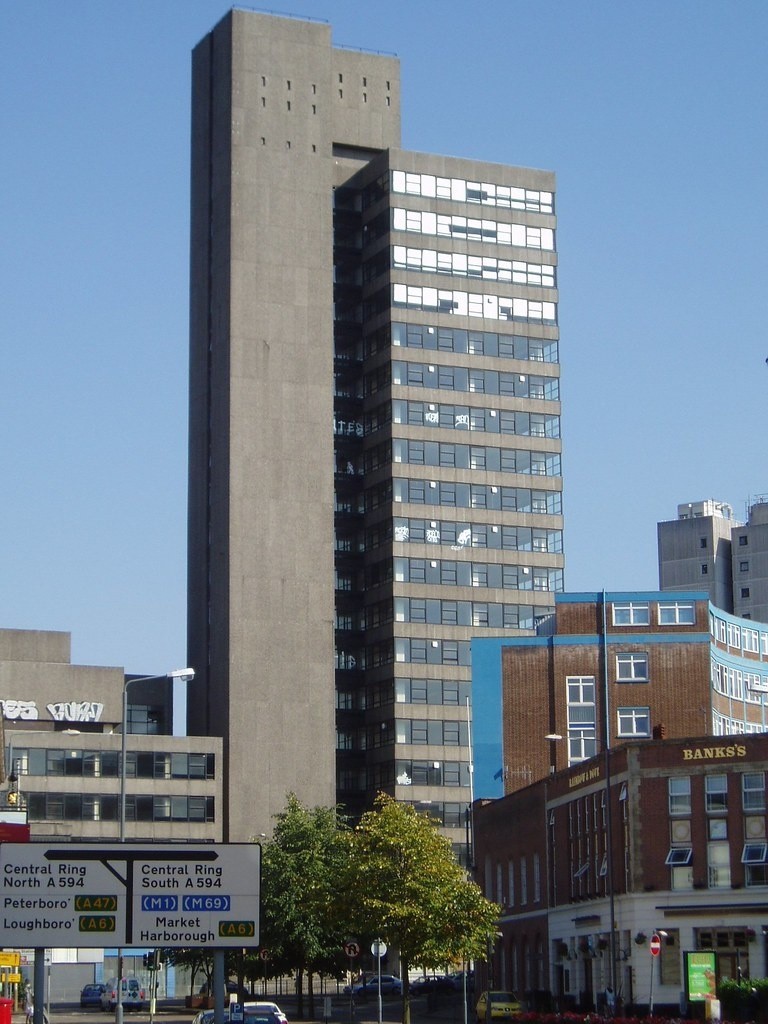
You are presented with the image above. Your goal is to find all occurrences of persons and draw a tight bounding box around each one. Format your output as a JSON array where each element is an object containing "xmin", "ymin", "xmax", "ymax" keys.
[
  {"xmin": 24, "ymin": 987, "xmax": 35, "ymax": 1024},
  {"xmin": 199, "ymin": 983, "xmax": 208, "ymax": 1009}
]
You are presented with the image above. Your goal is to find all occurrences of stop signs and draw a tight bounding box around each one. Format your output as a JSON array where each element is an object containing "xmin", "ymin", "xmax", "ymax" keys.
[{"xmin": 650, "ymin": 934, "xmax": 661, "ymax": 956}]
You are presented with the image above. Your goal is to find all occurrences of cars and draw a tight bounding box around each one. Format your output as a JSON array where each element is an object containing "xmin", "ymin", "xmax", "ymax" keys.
[
  {"xmin": 475, "ymin": 990, "xmax": 524, "ymax": 1024},
  {"xmin": 199, "ymin": 978, "xmax": 249, "ymax": 995},
  {"xmin": 79, "ymin": 983, "xmax": 107, "ymax": 1008},
  {"xmin": 243, "ymin": 1001, "xmax": 289, "ymax": 1024},
  {"xmin": 193, "ymin": 1008, "xmax": 254, "ymax": 1024},
  {"xmin": 344, "ymin": 970, "xmax": 475, "ymax": 999}
]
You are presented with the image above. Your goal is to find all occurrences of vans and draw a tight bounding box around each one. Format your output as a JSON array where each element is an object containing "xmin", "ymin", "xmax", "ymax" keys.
[{"xmin": 99, "ymin": 978, "xmax": 144, "ymax": 1011}]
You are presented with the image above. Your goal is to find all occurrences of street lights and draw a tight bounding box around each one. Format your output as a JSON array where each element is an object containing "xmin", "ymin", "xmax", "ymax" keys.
[
  {"xmin": 117, "ymin": 667, "xmax": 196, "ymax": 841},
  {"xmin": 486, "ymin": 930, "xmax": 504, "ymax": 1022},
  {"xmin": 545, "ymin": 734, "xmax": 618, "ymax": 1013}
]
